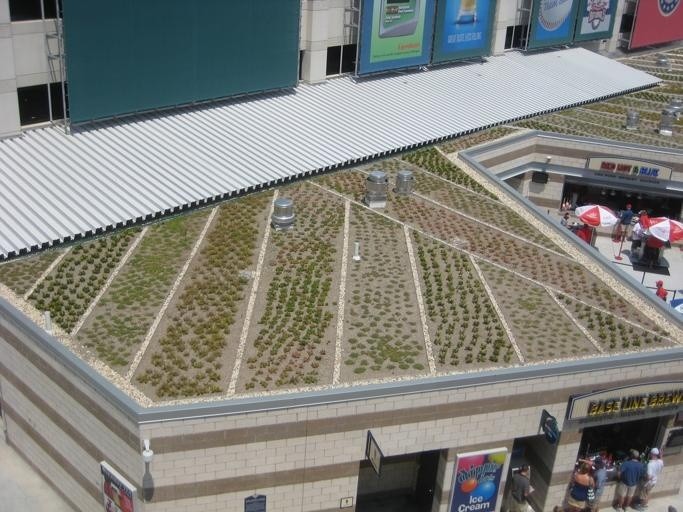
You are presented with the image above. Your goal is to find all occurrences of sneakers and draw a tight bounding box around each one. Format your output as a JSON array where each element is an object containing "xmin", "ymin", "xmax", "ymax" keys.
[{"xmin": 615, "ymin": 504, "xmax": 650, "ymax": 512}]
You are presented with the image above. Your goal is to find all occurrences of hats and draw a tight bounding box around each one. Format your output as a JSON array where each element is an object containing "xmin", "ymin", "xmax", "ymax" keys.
[
  {"xmin": 630, "ymin": 449, "xmax": 639, "ymax": 458},
  {"xmin": 651, "ymin": 447, "xmax": 659, "ymax": 455}
]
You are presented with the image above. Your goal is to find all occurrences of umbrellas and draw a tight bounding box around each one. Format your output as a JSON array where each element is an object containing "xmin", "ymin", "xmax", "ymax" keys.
[
  {"xmin": 575, "ymin": 205, "xmax": 618, "ymax": 246},
  {"xmin": 648, "ymin": 217, "xmax": 683, "ymax": 245}
]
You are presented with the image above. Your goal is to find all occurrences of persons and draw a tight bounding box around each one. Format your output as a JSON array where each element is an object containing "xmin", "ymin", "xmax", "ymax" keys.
[
  {"xmin": 560, "ymin": 213, "xmax": 570, "ymax": 228},
  {"xmin": 628, "ymin": 223, "xmax": 643, "ymax": 258},
  {"xmin": 509, "ymin": 464, "xmax": 533, "ymax": 512},
  {"xmin": 634, "ymin": 448, "xmax": 664, "ymax": 512},
  {"xmin": 619, "ymin": 204, "xmax": 633, "ymax": 242},
  {"xmin": 656, "ymin": 280, "xmax": 668, "ymax": 302},
  {"xmin": 568, "ymin": 463, "xmax": 595, "ymax": 512},
  {"xmin": 584, "ymin": 457, "xmax": 607, "ymax": 512},
  {"xmin": 614, "ymin": 451, "xmax": 644, "ymax": 512}
]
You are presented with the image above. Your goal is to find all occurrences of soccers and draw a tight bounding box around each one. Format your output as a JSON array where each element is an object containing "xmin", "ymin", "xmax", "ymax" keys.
[
  {"xmin": 461, "ymin": 479, "xmax": 477, "ymax": 492},
  {"xmin": 471, "ymin": 482, "xmax": 496, "ymax": 501},
  {"xmin": 458, "ymin": 455, "xmax": 484, "ymax": 474},
  {"xmin": 488, "ymin": 453, "xmax": 507, "ymax": 465}
]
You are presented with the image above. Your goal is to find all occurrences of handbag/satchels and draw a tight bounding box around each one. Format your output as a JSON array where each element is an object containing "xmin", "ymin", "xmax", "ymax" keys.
[{"xmin": 587, "ymin": 486, "xmax": 595, "ymax": 506}]
